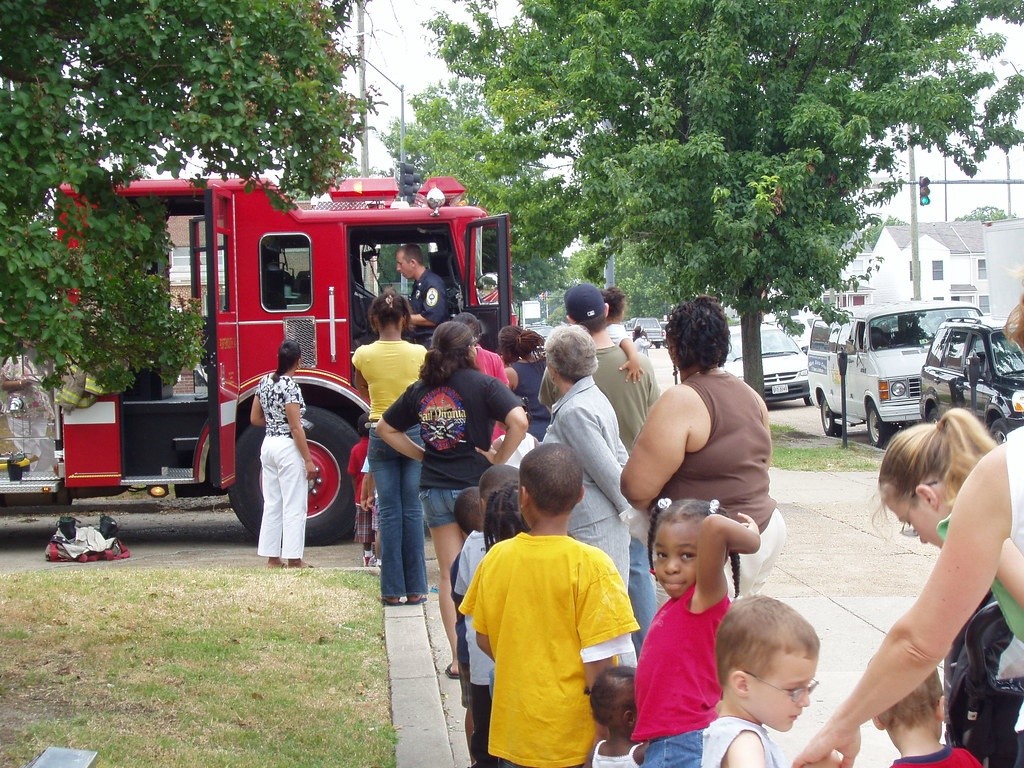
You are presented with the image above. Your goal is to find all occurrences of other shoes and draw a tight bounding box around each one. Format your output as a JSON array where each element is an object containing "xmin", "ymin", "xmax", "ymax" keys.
[{"xmin": 363, "ymin": 554, "xmax": 377, "ymax": 566}]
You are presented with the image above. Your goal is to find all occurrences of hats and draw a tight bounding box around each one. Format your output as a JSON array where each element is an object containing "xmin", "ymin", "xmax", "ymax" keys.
[{"xmin": 563, "ymin": 284, "xmax": 605, "ymax": 321}]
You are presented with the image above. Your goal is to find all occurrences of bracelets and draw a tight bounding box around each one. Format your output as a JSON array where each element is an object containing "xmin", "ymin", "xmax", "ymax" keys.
[{"xmin": 20, "ymin": 380, "xmax": 25, "ymax": 390}]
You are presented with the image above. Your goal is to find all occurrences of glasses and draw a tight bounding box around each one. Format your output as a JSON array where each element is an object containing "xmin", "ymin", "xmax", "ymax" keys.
[
  {"xmin": 741, "ymin": 671, "xmax": 818, "ymax": 702},
  {"xmin": 899, "ymin": 480, "xmax": 939, "ymax": 537}
]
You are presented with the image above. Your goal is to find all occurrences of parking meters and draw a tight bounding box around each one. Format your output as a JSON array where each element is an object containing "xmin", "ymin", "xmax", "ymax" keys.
[{"xmin": 837, "ymin": 353, "xmax": 848, "ymax": 448}]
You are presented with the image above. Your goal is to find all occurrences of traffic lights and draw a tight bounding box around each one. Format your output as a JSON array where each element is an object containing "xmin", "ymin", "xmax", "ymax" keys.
[{"xmin": 919, "ymin": 177, "xmax": 931, "ymax": 205}]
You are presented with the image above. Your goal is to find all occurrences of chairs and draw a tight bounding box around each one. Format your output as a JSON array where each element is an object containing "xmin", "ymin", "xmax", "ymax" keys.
[{"xmin": 861, "ymin": 327, "xmax": 884, "ymax": 349}]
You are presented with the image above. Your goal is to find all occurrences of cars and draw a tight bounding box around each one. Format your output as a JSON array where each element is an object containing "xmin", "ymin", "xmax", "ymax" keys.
[
  {"xmin": 723, "ymin": 314, "xmax": 815, "ymax": 406},
  {"xmin": 523, "ymin": 317, "xmax": 670, "ymax": 349}
]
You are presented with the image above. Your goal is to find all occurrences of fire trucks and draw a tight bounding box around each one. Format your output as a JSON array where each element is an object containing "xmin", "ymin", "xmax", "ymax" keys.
[{"xmin": 0, "ymin": 176, "xmax": 519, "ymax": 545}]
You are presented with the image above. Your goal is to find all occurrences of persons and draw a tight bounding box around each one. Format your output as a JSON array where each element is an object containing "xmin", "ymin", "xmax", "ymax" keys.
[
  {"xmin": 702, "ymin": 594, "xmax": 842, "ymax": 768},
  {"xmin": 250, "ymin": 340, "xmax": 317, "ymax": 569},
  {"xmin": 0, "ymin": 334, "xmax": 58, "ymax": 471},
  {"xmin": 349, "ymin": 243, "xmax": 786, "ymax": 768},
  {"xmin": 630, "ymin": 499, "xmax": 761, "ymax": 768},
  {"xmin": 791, "ymin": 292, "xmax": 1024, "ymax": 768}
]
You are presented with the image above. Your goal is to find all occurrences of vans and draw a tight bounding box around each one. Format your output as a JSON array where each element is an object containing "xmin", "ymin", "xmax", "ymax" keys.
[{"xmin": 807, "ymin": 299, "xmax": 982, "ymax": 448}]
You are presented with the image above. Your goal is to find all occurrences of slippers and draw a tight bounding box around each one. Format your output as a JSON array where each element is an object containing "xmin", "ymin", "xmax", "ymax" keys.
[
  {"xmin": 445, "ymin": 664, "xmax": 460, "ymax": 679},
  {"xmin": 381, "ymin": 596, "xmax": 427, "ymax": 605}
]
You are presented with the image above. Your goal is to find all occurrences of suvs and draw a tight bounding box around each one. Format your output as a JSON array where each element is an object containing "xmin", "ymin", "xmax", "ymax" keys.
[{"xmin": 919, "ymin": 314, "xmax": 1024, "ymax": 443}]
